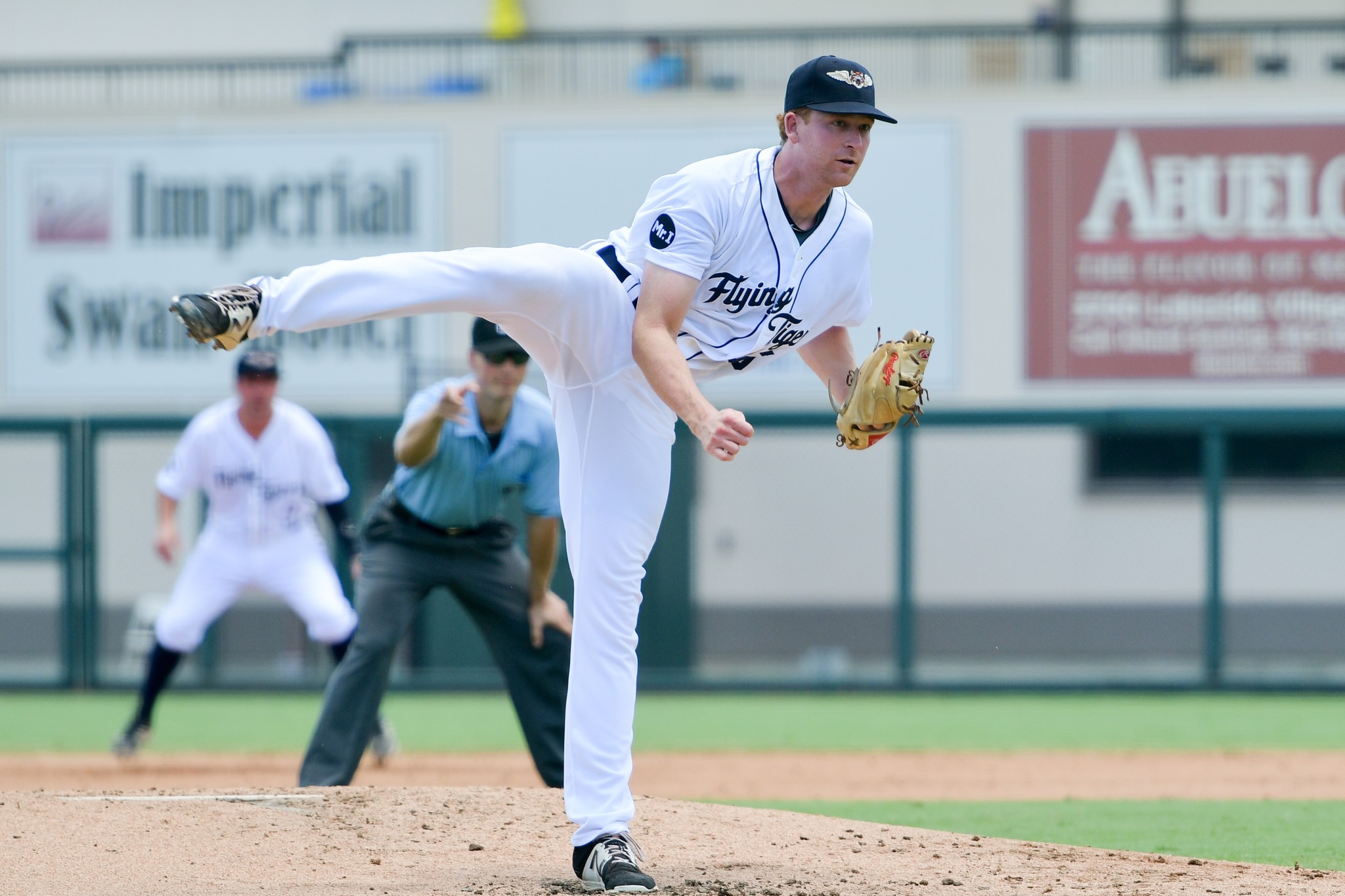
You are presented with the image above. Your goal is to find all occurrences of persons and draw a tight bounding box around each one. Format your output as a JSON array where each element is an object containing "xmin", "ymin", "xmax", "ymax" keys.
[
  {"xmin": 635, "ymin": 36, "xmax": 686, "ymax": 86},
  {"xmin": 171, "ymin": 56, "xmax": 934, "ymax": 894},
  {"xmin": 110, "ymin": 351, "xmax": 388, "ymax": 769},
  {"xmin": 299, "ymin": 316, "xmax": 572, "ymax": 788}
]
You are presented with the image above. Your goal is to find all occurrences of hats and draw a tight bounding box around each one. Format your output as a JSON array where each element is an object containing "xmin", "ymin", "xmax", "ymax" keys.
[
  {"xmin": 238, "ymin": 353, "xmax": 278, "ymax": 376},
  {"xmin": 473, "ymin": 316, "xmax": 519, "ymax": 346},
  {"xmin": 786, "ymin": 55, "xmax": 897, "ymax": 125}
]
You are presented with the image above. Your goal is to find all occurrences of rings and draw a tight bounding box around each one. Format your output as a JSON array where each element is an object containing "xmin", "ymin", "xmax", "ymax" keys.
[{"xmin": 441, "ymin": 396, "xmax": 449, "ymax": 408}]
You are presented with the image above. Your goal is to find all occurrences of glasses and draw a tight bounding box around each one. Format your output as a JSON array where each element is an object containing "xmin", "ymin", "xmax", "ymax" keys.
[{"xmin": 475, "ymin": 349, "xmax": 528, "ymax": 365}]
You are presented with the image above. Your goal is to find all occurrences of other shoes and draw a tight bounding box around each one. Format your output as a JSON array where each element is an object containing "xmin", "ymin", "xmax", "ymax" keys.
[
  {"xmin": 112, "ymin": 717, "xmax": 155, "ymax": 755},
  {"xmin": 368, "ymin": 714, "xmax": 390, "ymax": 763}
]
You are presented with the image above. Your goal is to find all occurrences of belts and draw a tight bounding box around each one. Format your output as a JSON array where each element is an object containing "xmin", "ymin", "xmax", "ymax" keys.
[
  {"xmin": 380, "ymin": 490, "xmax": 487, "ymax": 537},
  {"xmin": 598, "ymin": 246, "xmax": 640, "ymax": 310}
]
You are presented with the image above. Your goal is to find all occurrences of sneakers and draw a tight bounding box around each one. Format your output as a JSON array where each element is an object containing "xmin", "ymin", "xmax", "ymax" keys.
[
  {"xmin": 168, "ymin": 282, "xmax": 261, "ymax": 354},
  {"xmin": 573, "ymin": 832, "xmax": 656, "ymax": 893}
]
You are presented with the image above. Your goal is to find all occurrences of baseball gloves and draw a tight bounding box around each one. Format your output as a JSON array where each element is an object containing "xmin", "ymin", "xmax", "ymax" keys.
[{"xmin": 836, "ymin": 329, "xmax": 935, "ymax": 450}]
